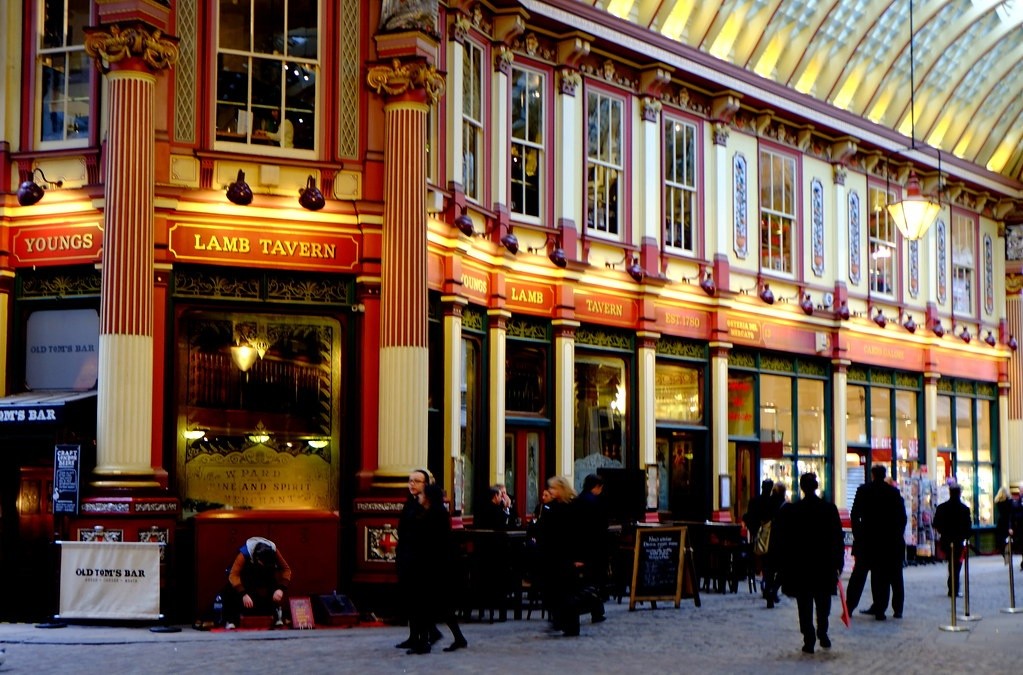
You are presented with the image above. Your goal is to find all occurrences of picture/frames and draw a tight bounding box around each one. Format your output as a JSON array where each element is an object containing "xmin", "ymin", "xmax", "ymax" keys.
[
  {"xmin": 645, "ymin": 464, "xmax": 660, "ymax": 511},
  {"xmin": 719, "ymin": 474, "xmax": 732, "ymax": 511}
]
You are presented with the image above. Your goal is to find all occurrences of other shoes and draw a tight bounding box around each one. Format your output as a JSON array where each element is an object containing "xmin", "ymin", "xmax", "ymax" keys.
[
  {"xmin": 225, "ymin": 621, "xmax": 235, "ymax": 629},
  {"xmin": 772, "ymin": 590, "xmax": 780, "ymax": 603},
  {"xmin": 767, "ymin": 602, "xmax": 774, "ymax": 608},
  {"xmin": 893, "ymin": 614, "xmax": 902, "ymax": 618},
  {"xmin": 428, "ymin": 631, "xmax": 443, "ymax": 645},
  {"xmin": 407, "ymin": 640, "xmax": 431, "ymax": 655},
  {"xmin": 817, "ymin": 628, "xmax": 831, "ymax": 648},
  {"xmin": 802, "ymin": 644, "xmax": 814, "ymax": 652},
  {"xmin": 396, "ymin": 638, "xmax": 419, "ymax": 649},
  {"xmin": 444, "ymin": 638, "xmax": 467, "ymax": 652},
  {"xmin": 275, "ymin": 620, "xmax": 283, "ymax": 625},
  {"xmin": 875, "ymin": 613, "xmax": 886, "ymax": 621}
]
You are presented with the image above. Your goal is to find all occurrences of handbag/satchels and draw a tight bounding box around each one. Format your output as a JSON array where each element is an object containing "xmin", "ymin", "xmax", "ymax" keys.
[{"xmin": 754, "ymin": 520, "xmax": 771, "ymax": 556}]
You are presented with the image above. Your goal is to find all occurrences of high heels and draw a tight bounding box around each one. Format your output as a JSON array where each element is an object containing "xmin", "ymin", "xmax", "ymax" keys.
[{"xmin": 860, "ymin": 609, "xmax": 875, "ymax": 615}]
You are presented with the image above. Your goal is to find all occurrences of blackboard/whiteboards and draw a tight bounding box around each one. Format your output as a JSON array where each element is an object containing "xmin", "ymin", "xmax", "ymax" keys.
[{"xmin": 631, "ymin": 525, "xmax": 697, "ymax": 601}]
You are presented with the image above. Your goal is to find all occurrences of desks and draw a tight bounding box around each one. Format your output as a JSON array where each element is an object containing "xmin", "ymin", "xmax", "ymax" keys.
[
  {"xmin": 217, "ymin": 132, "xmax": 270, "ymax": 140},
  {"xmin": 672, "ymin": 520, "xmax": 742, "ymax": 595}
]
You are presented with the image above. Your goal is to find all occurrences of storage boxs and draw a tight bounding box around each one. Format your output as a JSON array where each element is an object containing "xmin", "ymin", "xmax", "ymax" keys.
[{"xmin": 319, "ymin": 595, "xmax": 358, "ymax": 625}]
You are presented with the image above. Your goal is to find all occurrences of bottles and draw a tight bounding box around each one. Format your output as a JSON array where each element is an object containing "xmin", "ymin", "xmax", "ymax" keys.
[
  {"xmin": 93, "ymin": 525, "xmax": 104, "ymax": 541},
  {"xmin": 147, "ymin": 526, "xmax": 160, "ymax": 542},
  {"xmin": 214, "ymin": 595, "xmax": 222, "ymax": 622}
]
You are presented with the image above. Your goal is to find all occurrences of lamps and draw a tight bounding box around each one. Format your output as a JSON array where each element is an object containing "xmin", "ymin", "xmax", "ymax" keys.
[
  {"xmin": 682, "ymin": 270, "xmax": 1018, "ymax": 351},
  {"xmin": 226, "ymin": 169, "xmax": 253, "ymax": 205},
  {"xmin": 439, "ymin": 202, "xmax": 473, "ymax": 238},
  {"xmin": 886, "ymin": 0, "xmax": 942, "ymax": 240},
  {"xmin": 606, "ymin": 253, "xmax": 643, "ymax": 282},
  {"xmin": 528, "ymin": 238, "xmax": 566, "ymax": 267},
  {"xmin": 483, "ymin": 223, "xmax": 518, "ymax": 255},
  {"xmin": 17, "ymin": 168, "xmax": 62, "ymax": 206},
  {"xmin": 299, "ymin": 175, "xmax": 325, "ymax": 210}
]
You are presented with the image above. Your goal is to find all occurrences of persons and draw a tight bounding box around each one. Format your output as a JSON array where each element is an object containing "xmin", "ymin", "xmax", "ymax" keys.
[
  {"xmin": 223, "ymin": 536, "xmax": 291, "ymax": 628},
  {"xmin": 747, "ymin": 478, "xmax": 789, "ymax": 603},
  {"xmin": 844, "ymin": 465, "xmax": 907, "ymax": 621},
  {"xmin": 773, "ymin": 472, "xmax": 844, "ymax": 653},
  {"xmin": 255, "ymin": 109, "xmax": 294, "ymax": 148},
  {"xmin": 994, "ymin": 485, "xmax": 1023, "ymax": 555},
  {"xmin": 474, "ymin": 485, "xmax": 520, "ymax": 530},
  {"xmin": 528, "ymin": 473, "xmax": 609, "ymax": 638},
  {"xmin": 931, "ymin": 487, "xmax": 971, "ymax": 598},
  {"xmin": 395, "ymin": 468, "xmax": 467, "ymax": 654}
]
